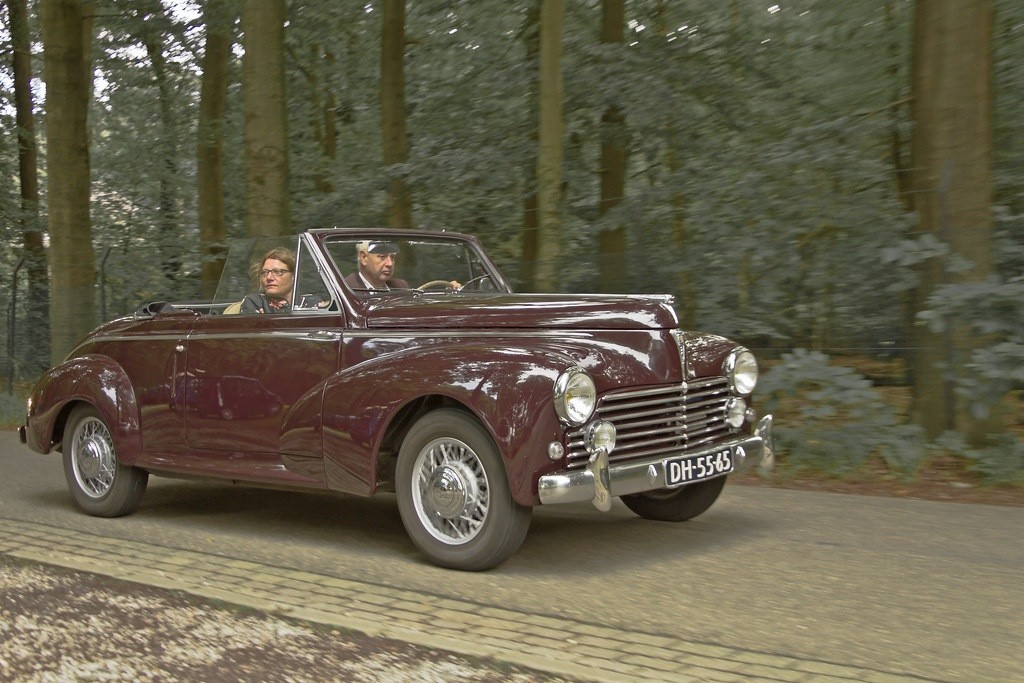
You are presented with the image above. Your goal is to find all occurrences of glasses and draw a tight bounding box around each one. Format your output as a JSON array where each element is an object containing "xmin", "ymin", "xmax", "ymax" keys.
[{"xmin": 260, "ymin": 268, "xmax": 292, "ymax": 277}]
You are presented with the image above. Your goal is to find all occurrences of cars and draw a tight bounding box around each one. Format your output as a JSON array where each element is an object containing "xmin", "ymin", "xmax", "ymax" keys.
[{"xmin": 17, "ymin": 226, "xmax": 777, "ymax": 574}]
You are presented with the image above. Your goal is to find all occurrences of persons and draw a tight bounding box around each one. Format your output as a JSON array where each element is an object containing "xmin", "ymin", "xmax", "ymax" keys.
[
  {"xmin": 223, "ymin": 246, "xmax": 330, "ymax": 314},
  {"xmin": 342, "ymin": 239, "xmax": 464, "ymax": 291}
]
